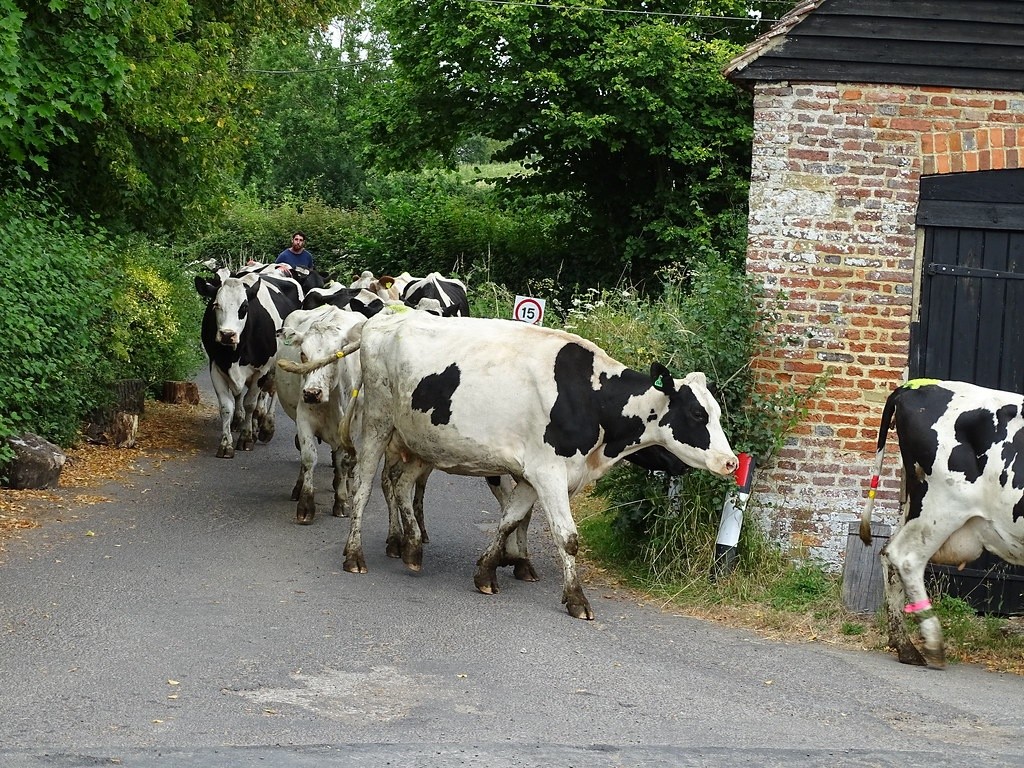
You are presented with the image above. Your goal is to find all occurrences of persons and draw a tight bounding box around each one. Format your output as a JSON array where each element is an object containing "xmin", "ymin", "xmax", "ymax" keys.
[{"xmin": 275, "ymin": 232, "xmax": 313, "ymax": 274}]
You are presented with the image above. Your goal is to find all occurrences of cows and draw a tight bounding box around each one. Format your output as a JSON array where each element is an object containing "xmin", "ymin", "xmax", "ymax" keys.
[
  {"xmin": 201, "ymin": 257, "xmax": 470, "ymax": 445},
  {"xmin": 272, "ymin": 301, "xmax": 370, "ymax": 526},
  {"xmin": 192, "ymin": 273, "xmax": 304, "ymax": 459},
  {"xmin": 857, "ymin": 376, "xmax": 1024, "ymax": 672},
  {"xmin": 272, "ymin": 302, "xmax": 741, "ymax": 621}
]
